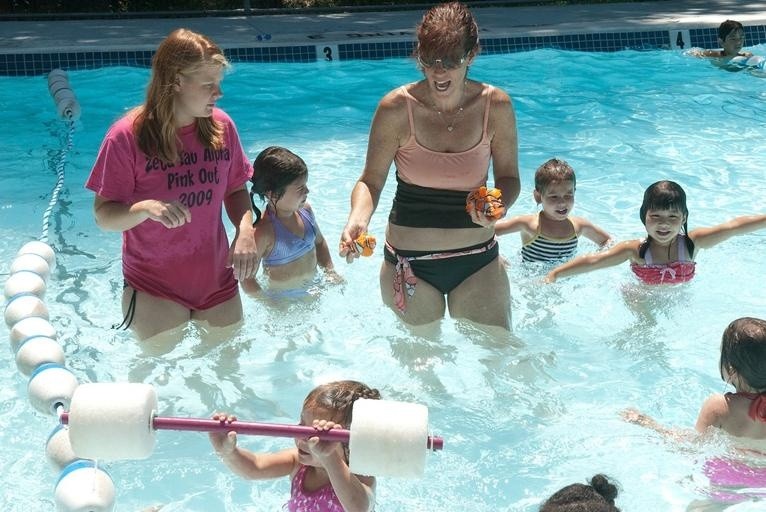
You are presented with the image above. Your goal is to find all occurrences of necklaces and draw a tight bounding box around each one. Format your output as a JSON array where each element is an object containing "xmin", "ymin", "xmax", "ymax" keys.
[{"xmin": 426, "ymin": 80, "xmax": 469, "ymax": 133}]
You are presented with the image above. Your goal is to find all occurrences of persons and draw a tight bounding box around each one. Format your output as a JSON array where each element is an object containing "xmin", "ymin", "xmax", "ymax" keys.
[
  {"xmin": 334, "ymin": 2, "xmax": 525, "ymax": 402},
  {"xmin": 237, "ymin": 145, "xmax": 348, "ymax": 313},
  {"xmin": 692, "ymin": 20, "xmax": 766, "ymax": 81},
  {"xmin": 538, "ymin": 472, "xmax": 618, "ymax": 512},
  {"xmin": 547, "ymin": 180, "xmax": 766, "ymax": 348},
  {"xmin": 618, "ymin": 315, "xmax": 765, "ymax": 512},
  {"xmin": 84, "ymin": 27, "xmax": 259, "ymax": 384},
  {"xmin": 495, "ymin": 157, "xmax": 615, "ymax": 265},
  {"xmin": 205, "ymin": 378, "xmax": 382, "ymax": 511}
]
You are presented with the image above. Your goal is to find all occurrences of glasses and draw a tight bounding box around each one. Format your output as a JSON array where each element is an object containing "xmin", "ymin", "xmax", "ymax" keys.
[{"xmin": 418, "ymin": 47, "xmax": 472, "ymax": 72}]
